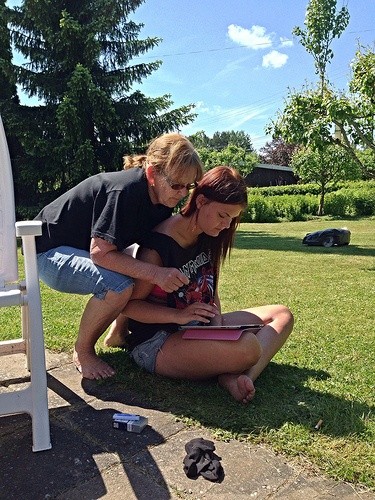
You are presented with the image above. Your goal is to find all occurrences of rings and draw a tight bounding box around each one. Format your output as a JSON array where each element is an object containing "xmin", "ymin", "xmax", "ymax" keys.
[{"xmin": 193, "ymin": 308, "xmax": 196, "ymax": 315}]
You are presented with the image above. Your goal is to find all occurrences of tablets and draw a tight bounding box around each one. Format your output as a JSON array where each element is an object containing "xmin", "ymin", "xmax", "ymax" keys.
[{"xmin": 180, "ymin": 324, "xmax": 264, "ymax": 330}]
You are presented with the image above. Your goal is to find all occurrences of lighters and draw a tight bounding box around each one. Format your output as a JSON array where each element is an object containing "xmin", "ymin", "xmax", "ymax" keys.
[{"xmin": 114, "ymin": 413, "xmax": 139, "ymax": 420}]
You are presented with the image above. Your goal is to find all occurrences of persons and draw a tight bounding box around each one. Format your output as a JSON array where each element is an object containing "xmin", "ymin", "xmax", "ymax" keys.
[
  {"xmin": 21, "ymin": 134, "xmax": 204, "ymax": 380},
  {"xmin": 122, "ymin": 166, "xmax": 295, "ymax": 403}
]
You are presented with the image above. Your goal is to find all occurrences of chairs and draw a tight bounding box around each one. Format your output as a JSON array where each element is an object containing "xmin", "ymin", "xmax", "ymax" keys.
[{"xmin": 0, "ymin": 116, "xmax": 52, "ymax": 452}]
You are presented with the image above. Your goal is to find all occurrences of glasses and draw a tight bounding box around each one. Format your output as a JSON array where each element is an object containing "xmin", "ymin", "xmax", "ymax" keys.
[{"xmin": 153, "ymin": 165, "xmax": 198, "ymax": 190}]
